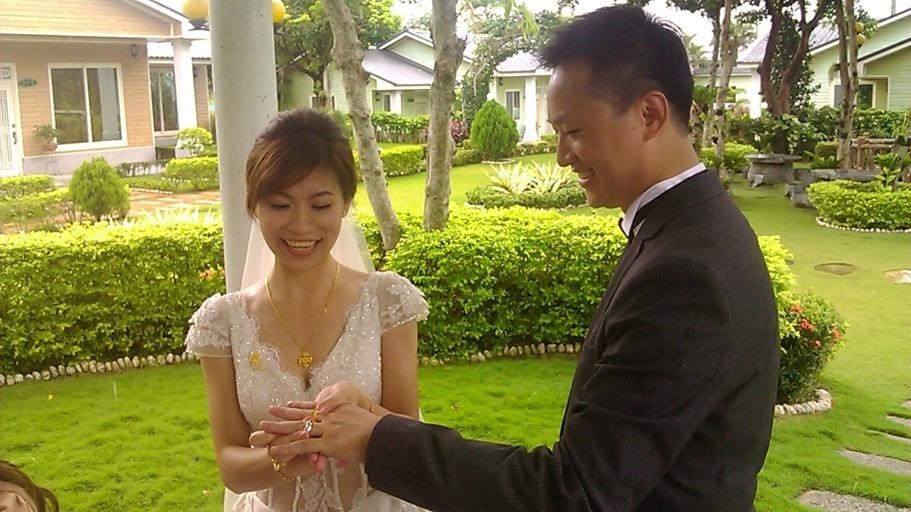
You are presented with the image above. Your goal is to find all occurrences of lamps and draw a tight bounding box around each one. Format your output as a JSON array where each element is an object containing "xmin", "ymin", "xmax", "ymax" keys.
[{"xmin": 130, "ymin": 44, "xmax": 139, "ymax": 59}]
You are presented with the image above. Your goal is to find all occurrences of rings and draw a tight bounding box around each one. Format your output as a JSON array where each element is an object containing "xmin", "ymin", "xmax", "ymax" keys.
[
  {"xmin": 312, "ymin": 408, "xmax": 320, "ymax": 419},
  {"xmin": 304, "ymin": 420, "xmax": 313, "ymax": 433}
]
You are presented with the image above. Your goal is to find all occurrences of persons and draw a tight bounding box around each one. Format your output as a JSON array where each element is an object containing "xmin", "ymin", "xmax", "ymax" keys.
[
  {"xmin": 256, "ymin": 3, "xmax": 782, "ymax": 511},
  {"xmin": 183, "ymin": 105, "xmax": 432, "ymax": 512}
]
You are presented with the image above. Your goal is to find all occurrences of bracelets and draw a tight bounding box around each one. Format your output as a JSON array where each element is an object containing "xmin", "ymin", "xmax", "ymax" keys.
[
  {"xmin": 368, "ymin": 396, "xmax": 374, "ymax": 413},
  {"xmin": 271, "ymin": 458, "xmax": 296, "ymax": 482}
]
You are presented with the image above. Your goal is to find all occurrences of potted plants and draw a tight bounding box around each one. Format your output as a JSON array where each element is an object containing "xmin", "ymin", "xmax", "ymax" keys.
[{"xmin": 33, "ymin": 121, "xmax": 67, "ymax": 151}]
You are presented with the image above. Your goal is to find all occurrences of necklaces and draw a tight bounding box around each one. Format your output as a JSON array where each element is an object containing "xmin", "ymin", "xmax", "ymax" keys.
[{"xmin": 264, "ymin": 261, "xmax": 341, "ymax": 369}]
